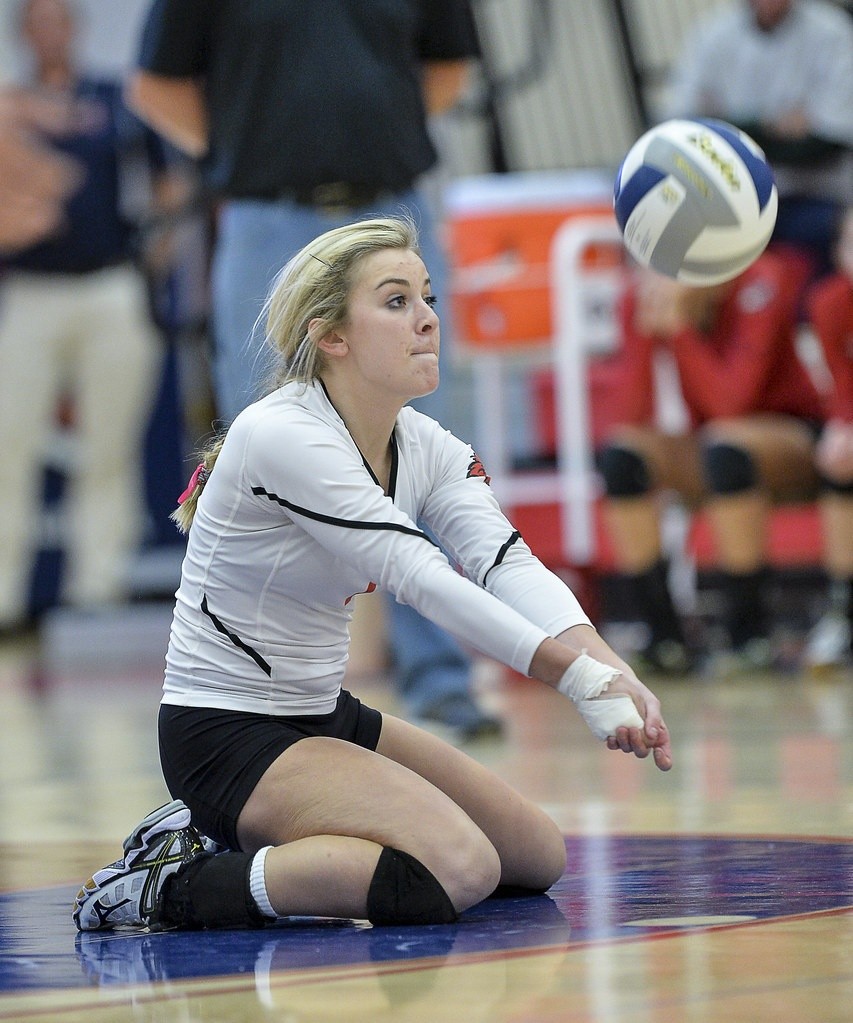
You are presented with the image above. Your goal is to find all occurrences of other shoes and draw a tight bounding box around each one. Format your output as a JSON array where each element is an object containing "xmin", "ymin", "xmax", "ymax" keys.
[
  {"xmin": 808, "ymin": 615, "xmax": 850, "ymax": 666},
  {"xmin": 644, "ymin": 623, "xmax": 704, "ymax": 673},
  {"xmin": 731, "ymin": 623, "xmax": 781, "ymax": 666}
]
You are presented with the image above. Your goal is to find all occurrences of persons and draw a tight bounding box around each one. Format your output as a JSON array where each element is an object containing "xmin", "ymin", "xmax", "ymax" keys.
[
  {"xmin": 71, "ymin": 212, "xmax": 672, "ymax": 934},
  {"xmin": 0, "ymin": 0, "xmax": 852, "ymax": 743}
]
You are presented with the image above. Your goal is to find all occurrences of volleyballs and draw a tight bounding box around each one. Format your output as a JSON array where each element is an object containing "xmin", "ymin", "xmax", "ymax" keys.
[{"xmin": 613, "ymin": 116, "xmax": 778, "ymax": 286}]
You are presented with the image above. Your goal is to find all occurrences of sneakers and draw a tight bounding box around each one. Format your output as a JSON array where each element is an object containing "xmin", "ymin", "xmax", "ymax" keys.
[
  {"xmin": 73, "ymin": 799, "xmax": 208, "ymax": 935},
  {"xmin": 197, "ymin": 831, "xmax": 230, "ymax": 855}
]
